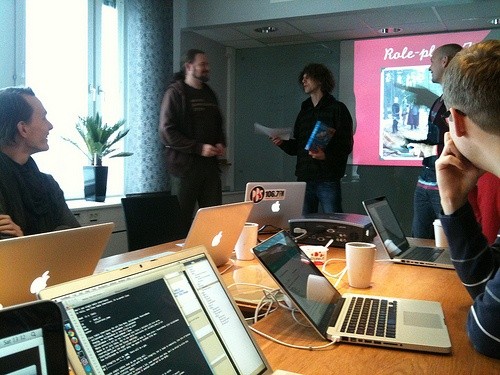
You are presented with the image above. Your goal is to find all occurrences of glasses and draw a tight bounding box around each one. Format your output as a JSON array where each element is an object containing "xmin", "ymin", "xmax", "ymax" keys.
[{"xmin": 440, "ymin": 108, "xmax": 466, "ymax": 127}]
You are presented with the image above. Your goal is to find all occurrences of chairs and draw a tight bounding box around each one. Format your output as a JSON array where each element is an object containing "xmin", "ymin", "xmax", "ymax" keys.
[{"xmin": 120, "ymin": 194, "xmax": 187, "ymax": 252}]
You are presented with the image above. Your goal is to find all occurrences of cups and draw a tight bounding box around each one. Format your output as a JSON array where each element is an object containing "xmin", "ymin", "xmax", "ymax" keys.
[
  {"xmin": 235, "ymin": 222, "xmax": 259, "ymax": 260},
  {"xmin": 345, "ymin": 242, "xmax": 376, "ymax": 289},
  {"xmin": 300, "ymin": 245, "xmax": 329, "ymax": 273},
  {"xmin": 432, "ymin": 219, "xmax": 448, "ymax": 248}
]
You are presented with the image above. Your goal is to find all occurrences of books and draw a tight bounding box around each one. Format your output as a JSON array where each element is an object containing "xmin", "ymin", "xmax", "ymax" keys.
[{"xmin": 304, "ymin": 121, "xmax": 335, "ymax": 153}]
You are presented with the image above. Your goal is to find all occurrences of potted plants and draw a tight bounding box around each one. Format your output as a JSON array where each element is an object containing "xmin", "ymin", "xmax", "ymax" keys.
[{"xmin": 61, "ymin": 113, "xmax": 134, "ymax": 202}]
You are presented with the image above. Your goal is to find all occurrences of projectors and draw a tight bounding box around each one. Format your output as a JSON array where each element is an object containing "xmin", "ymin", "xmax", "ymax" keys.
[{"xmin": 285, "ymin": 213, "xmax": 376, "ymax": 247}]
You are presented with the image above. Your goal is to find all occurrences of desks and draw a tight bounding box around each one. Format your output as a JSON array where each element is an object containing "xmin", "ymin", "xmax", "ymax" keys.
[{"xmin": 93, "ymin": 236, "xmax": 500, "ymax": 375}]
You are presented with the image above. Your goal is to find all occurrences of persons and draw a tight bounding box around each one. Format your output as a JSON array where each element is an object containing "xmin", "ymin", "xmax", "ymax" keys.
[
  {"xmin": 158, "ymin": 50, "xmax": 228, "ymax": 234},
  {"xmin": 383, "ymin": 44, "xmax": 464, "ymax": 240},
  {"xmin": 468, "ymin": 171, "xmax": 500, "ymax": 247},
  {"xmin": 435, "ymin": 40, "xmax": 500, "ymax": 360},
  {"xmin": 392, "ymin": 96, "xmax": 419, "ymax": 134},
  {"xmin": 0, "ymin": 87, "xmax": 81, "ymax": 240},
  {"xmin": 268, "ymin": 63, "xmax": 353, "ymax": 213}
]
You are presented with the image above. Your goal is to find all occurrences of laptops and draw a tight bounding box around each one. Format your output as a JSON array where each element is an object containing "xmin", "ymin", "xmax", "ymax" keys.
[
  {"xmin": 0, "ymin": 300, "xmax": 70, "ymax": 375},
  {"xmin": 140, "ymin": 201, "xmax": 252, "ymax": 267},
  {"xmin": 362, "ymin": 195, "xmax": 456, "ymax": 273},
  {"xmin": 251, "ymin": 230, "xmax": 453, "ymax": 354},
  {"xmin": 37, "ymin": 246, "xmax": 304, "ymax": 375},
  {"xmin": 0, "ymin": 221, "xmax": 114, "ymax": 307},
  {"xmin": 244, "ymin": 182, "xmax": 306, "ymax": 233}
]
